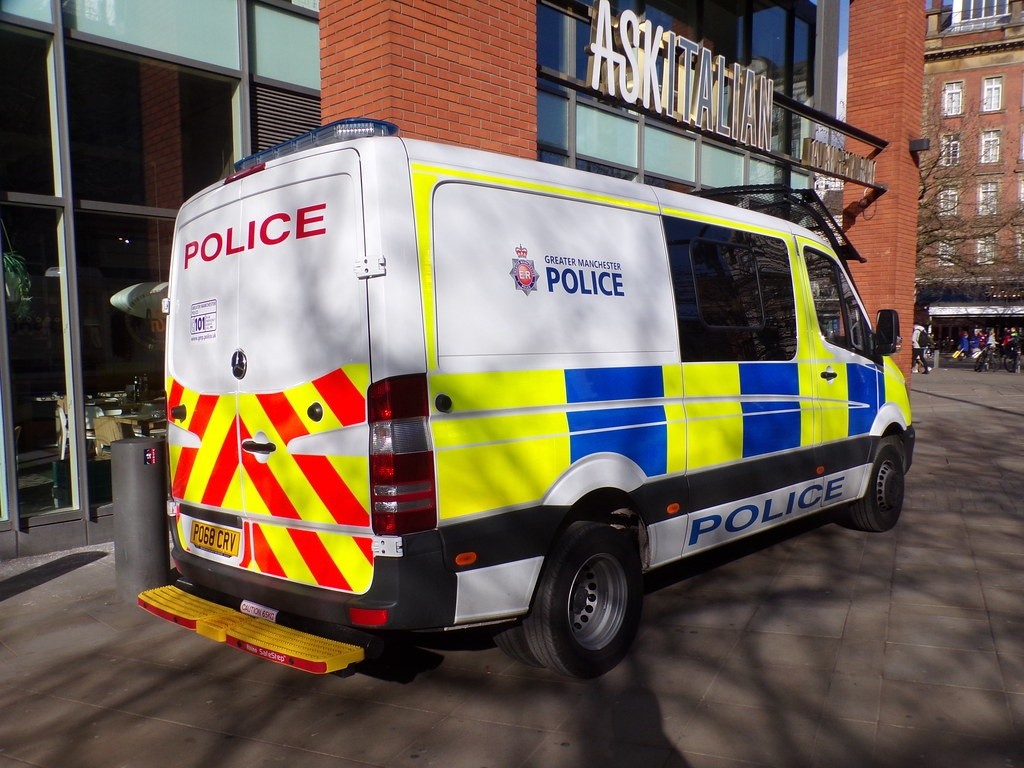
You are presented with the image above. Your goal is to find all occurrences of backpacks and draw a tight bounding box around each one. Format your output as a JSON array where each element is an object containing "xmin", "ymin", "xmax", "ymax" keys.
[{"xmin": 914, "ymin": 329, "xmax": 930, "ymax": 348}]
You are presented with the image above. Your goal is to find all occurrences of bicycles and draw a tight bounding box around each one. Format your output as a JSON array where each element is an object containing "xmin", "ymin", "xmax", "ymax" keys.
[{"xmin": 974, "ymin": 342, "xmax": 1020, "ymax": 373}]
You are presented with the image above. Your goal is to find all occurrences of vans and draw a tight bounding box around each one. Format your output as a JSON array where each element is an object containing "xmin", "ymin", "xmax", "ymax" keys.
[{"xmin": 135, "ymin": 117, "xmax": 917, "ymax": 677}]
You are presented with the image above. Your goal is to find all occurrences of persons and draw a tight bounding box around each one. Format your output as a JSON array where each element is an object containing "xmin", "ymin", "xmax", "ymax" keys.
[{"xmin": 912, "ymin": 321, "xmax": 1024, "ymax": 374}]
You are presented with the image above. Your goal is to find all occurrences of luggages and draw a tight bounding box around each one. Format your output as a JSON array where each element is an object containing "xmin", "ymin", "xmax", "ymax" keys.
[{"xmin": 952, "ymin": 348, "xmax": 964, "ymax": 358}]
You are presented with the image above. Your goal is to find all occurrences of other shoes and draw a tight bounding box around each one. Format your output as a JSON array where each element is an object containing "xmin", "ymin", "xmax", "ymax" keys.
[
  {"xmin": 922, "ymin": 370, "xmax": 928, "ymax": 374},
  {"xmin": 912, "ymin": 369, "xmax": 918, "ymax": 373}
]
